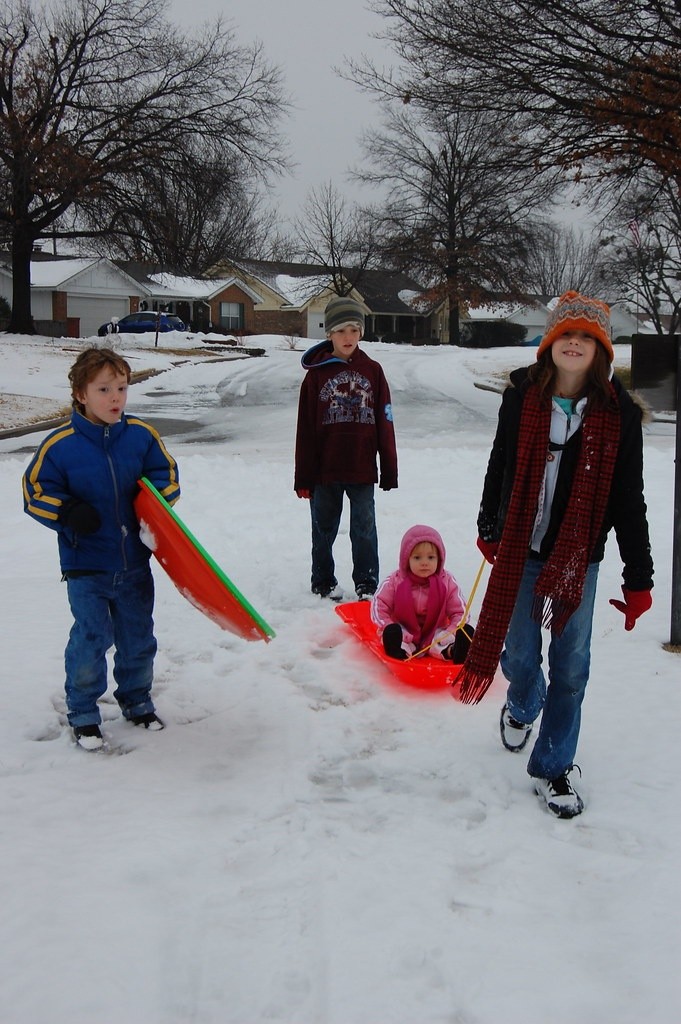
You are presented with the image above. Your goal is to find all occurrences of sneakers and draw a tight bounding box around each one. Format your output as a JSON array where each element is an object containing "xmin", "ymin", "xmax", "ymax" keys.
[
  {"xmin": 501, "ymin": 701, "xmax": 533, "ymax": 753},
  {"xmin": 533, "ymin": 774, "xmax": 585, "ymax": 820}
]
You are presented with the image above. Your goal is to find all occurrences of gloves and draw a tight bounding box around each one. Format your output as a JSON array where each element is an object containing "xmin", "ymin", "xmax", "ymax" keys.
[
  {"xmin": 60, "ymin": 500, "xmax": 103, "ymax": 536},
  {"xmin": 477, "ymin": 536, "xmax": 500, "ymax": 566},
  {"xmin": 610, "ymin": 585, "xmax": 652, "ymax": 632},
  {"xmin": 296, "ymin": 487, "xmax": 312, "ymax": 499},
  {"xmin": 379, "ymin": 484, "xmax": 391, "ymax": 492}
]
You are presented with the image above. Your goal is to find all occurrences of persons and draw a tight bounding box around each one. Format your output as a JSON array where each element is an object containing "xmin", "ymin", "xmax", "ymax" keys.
[
  {"xmin": 371, "ymin": 524, "xmax": 475, "ymax": 664},
  {"xmin": 293, "ymin": 297, "xmax": 399, "ymax": 602},
  {"xmin": 105, "ymin": 316, "xmax": 120, "ymax": 352},
  {"xmin": 477, "ymin": 291, "xmax": 654, "ymax": 819},
  {"xmin": 20, "ymin": 346, "xmax": 182, "ymax": 750}
]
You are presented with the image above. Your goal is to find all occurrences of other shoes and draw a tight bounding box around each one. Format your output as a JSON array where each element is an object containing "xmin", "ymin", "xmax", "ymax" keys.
[
  {"xmin": 73, "ymin": 725, "xmax": 103, "ymax": 752},
  {"xmin": 125, "ymin": 712, "xmax": 164, "ymax": 733},
  {"xmin": 453, "ymin": 624, "xmax": 474, "ymax": 664},
  {"xmin": 383, "ymin": 623, "xmax": 405, "ymax": 659},
  {"xmin": 354, "ymin": 586, "xmax": 375, "ymax": 601},
  {"xmin": 312, "ymin": 580, "xmax": 343, "ymax": 603}
]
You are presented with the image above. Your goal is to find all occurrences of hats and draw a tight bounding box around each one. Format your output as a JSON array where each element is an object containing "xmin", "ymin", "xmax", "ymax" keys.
[
  {"xmin": 324, "ymin": 296, "xmax": 364, "ymax": 338},
  {"xmin": 538, "ymin": 291, "xmax": 614, "ymax": 366}
]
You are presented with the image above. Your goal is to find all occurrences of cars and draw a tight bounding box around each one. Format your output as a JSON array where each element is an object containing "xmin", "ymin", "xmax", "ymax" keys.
[{"xmin": 98, "ymin": 311, "xmax": 185, "ymax": 335}]
